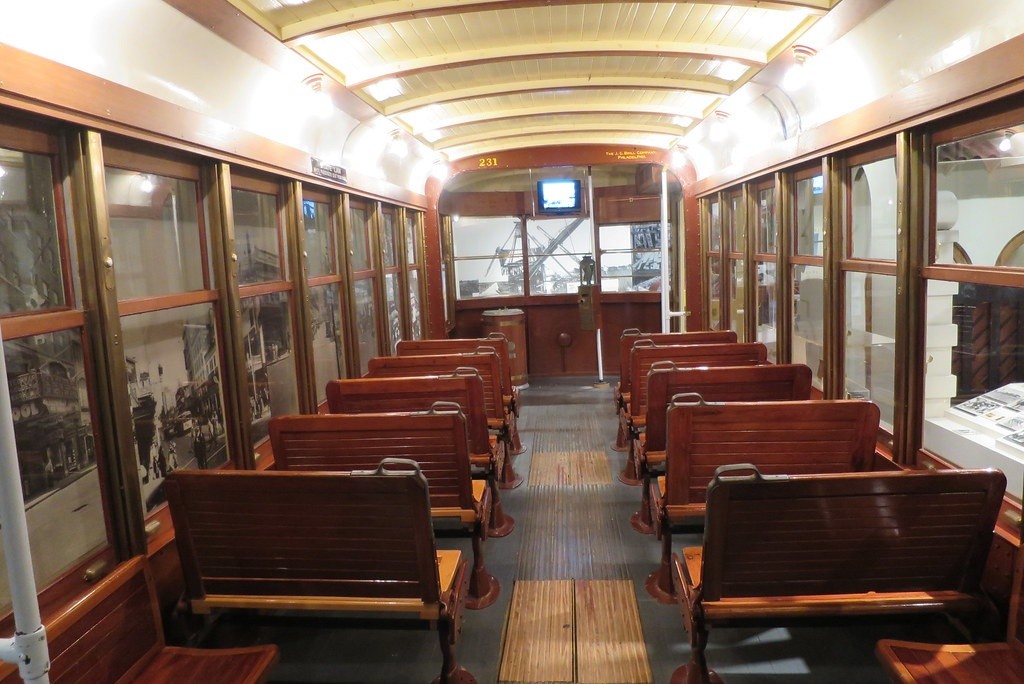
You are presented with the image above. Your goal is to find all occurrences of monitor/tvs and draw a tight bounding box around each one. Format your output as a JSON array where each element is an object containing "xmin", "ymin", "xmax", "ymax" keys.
[{"xmin": 537, "ymin": 180, "xmax": 581, "ymax": 213}]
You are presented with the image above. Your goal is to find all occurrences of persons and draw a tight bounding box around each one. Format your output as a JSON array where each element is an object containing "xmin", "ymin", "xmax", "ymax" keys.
[
  {"xmin": 249, "ymin": 388, "xmax": 269, "ymax": 422},
  {"xmin": 153, "ymin": 410, "xmax": 219, "ymax": 478}
]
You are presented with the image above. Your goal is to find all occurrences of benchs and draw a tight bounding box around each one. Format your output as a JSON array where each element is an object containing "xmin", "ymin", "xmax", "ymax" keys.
[
  {"xmin": 669, "ymin": 463, "xmax": 1007, "ymax": 684},
  {"xmin": 396, "ymin": 332, "xmax": 527, "ymax": 455},
  {"xmin": 269, "ymin": 401, "xmax": 500, "ymax": 609},
  {"xmin": 367, "ymin": 345, "xmax": 525, "ymax": 489},
  {"xmin": 325, "ymin": 366, "xmax": 515, "ymax": 537},
  {"xmin": 644, "ymin": 392, "xmax": 881, "ymax": 606},
  {"xmin": 875, "ymin": 469, "xmax": 1024, "ymax": 684},
  {"xmin": 165, "ymin": 457, "xmax": 476, "ymax": 684},
  {"xmin": 631, "ymin": 360, "xmax": 812, "ymax": 532},
  {"xmin": 0, "ymin": 555, "xmax": 281, "ymax": 684},
  {"xmin": 610, "ymin": 328, "xmax": 738, "ymax": 452},
  {"xmin": 616, "ymin": 339, "xmax": 767, "ymax": 485}
]
